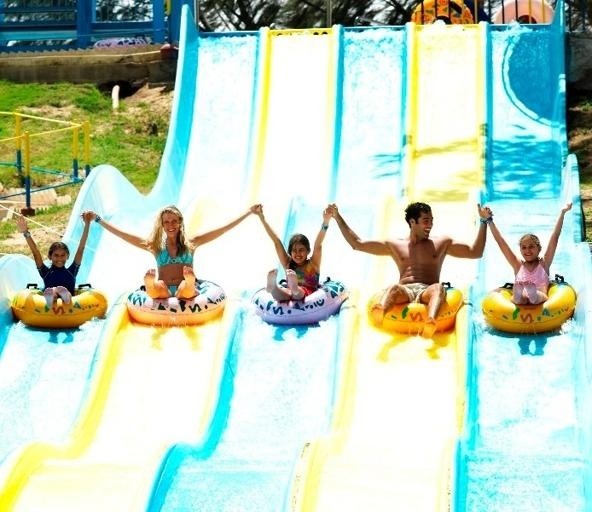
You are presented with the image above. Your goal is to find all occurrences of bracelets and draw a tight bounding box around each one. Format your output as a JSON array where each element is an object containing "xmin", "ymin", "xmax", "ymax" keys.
[
  {"xmin": 480, "ymin": 218, "xmax": 486, "ymax": 224},
  {"xmin": 15, "ymin": 209, "xmax": 91, "ymax": 309},
  {"xmin": 484, "ymin": 217, "xmax": 492, "ymax": 224},
  {"xmin": 24, "ymin": 231, "xmax": 31, "ymax": 238},
  {"xmin": 95, "ymin": 215, "xmax": 100, "ymax": 222},
  {"xmin": 322, "ymin": 224, "xmax": 328, "ymax": 230}
]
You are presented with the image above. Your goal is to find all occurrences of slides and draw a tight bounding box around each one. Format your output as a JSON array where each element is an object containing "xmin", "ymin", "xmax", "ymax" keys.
[{"xmin": 1, "ymin": 3, "xmax": 591, "ymax": 507}]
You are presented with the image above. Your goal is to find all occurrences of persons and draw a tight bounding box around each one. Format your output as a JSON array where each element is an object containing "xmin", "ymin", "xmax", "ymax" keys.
[
  {"xmin": 81, "ymin": 203, "xmax": 263, "ymax": 298},
  {"xmin": 329, "ymin": 204, "xmax": 494, "ymax": 341},
  {"xmin": 481, "ymin": 202, "xmax": 572, "ymax": 305},
  {"xmin": 256, "ymin": 205, "xmax": 332, "ymax": 301}
]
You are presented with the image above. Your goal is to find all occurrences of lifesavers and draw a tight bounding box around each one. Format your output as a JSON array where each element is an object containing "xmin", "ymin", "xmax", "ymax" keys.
[
  {"xmin": 127, "ymin": 280, "xmax": 225, "ymax": 325},
  {"xmin": 12, "ymin": 283, "xmax": 105, "ymax": 326},
  {"xmin": 481, "ymin": 280, "xmax": 576, "ymax": 333},
  {"xmin": 411, "ymin": 0, "xmax": 473, "ymax": 25},
  {"xmin": 494, "ymin": 0, "xmax": 553, "ymax": 24},
  {"xmin": 367, "ymin": 283, "xmax": 465, "ymax": 334},
  {"xmin": 252, "ymin": 280, "xmax": 346, "ymax": 325}
]
